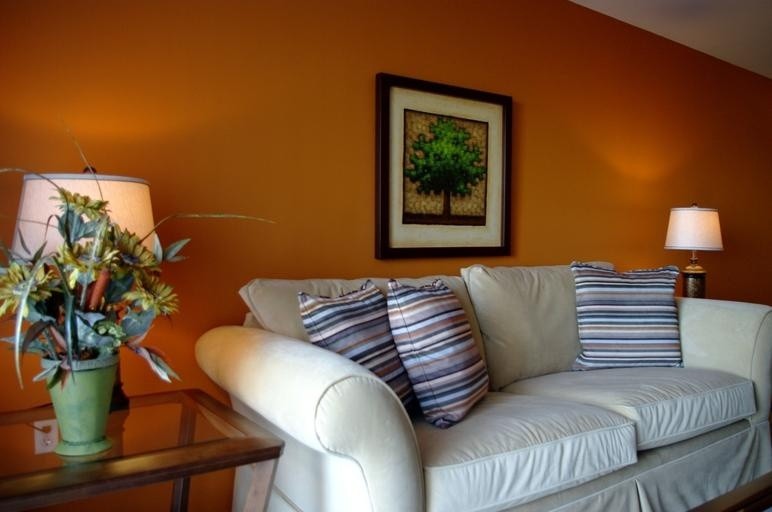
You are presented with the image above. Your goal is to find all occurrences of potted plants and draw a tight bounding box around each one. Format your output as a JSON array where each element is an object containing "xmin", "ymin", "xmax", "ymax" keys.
[{"xmin": 0, "ymin": 138, "xmax": 277, "ymax": 456}]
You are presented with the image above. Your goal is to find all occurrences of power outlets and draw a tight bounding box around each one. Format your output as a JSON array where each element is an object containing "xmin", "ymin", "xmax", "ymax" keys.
[{"xmin": 33, "ymin": 420, "xmax": 59, "ymax": 454}]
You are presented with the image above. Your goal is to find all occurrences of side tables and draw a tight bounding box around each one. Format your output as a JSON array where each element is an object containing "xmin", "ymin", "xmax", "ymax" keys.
[{"xmin": 1, "ymin": 389, "xmax": 285, "ymax": 511}]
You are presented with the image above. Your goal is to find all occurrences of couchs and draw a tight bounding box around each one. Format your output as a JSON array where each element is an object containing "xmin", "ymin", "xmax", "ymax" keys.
[{"xmin": 194, "ymin": 261, "xmax": 771, "ymax": 511}]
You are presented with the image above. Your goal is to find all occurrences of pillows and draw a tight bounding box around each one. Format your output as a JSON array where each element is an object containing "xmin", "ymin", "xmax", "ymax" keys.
[
  {"xmin": 568, "ymin": 257, "xmax": 684, "ymax": 372},
  {"xmin": 296, "ymin": 276, "xmax": 493, "ymax": 430}
]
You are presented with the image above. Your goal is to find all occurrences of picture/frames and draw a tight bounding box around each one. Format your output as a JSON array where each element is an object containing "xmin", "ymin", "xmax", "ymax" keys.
[{"xmin": 371, "ymin": 69, "xmax": 515, "ymax": 259}]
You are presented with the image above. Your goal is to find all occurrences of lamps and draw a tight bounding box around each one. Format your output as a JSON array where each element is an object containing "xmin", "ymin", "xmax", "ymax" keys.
[
  {"xmin": 663, "ymin": 202, "xmax": 726, "ymax": 300},
  {"xmin": 12, "ymin": 163, "xmax": 158, "ymax": 411}
]
